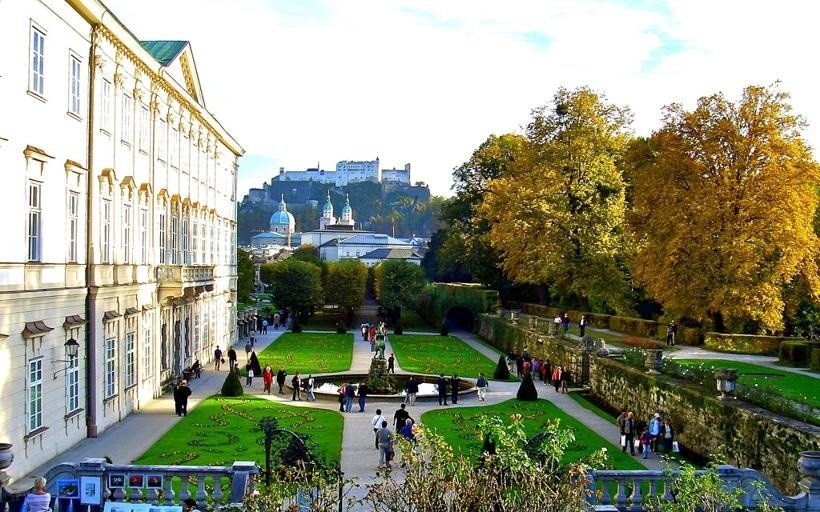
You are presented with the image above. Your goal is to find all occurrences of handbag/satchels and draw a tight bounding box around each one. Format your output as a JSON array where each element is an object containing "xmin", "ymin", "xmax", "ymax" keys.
[
  {"xmin": 634, "ymin": 439, "xmax": 640, "ymax": 448},
  {"xmin": 673, "ymin": 441, "xmax": 680, "ymax": 453},
  {"xmin": 620, "ymin": 435, "xmax": 626, "ymax": 447}
]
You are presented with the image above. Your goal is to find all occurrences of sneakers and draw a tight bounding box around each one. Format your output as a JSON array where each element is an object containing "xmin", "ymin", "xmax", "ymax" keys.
[
  {"xmin": 632, "ymin": 452, "xmax": 670, "ymax": 459},
  {"xmin": 376, "ymin": 462, "xmax": 406, "ymax": 471}
]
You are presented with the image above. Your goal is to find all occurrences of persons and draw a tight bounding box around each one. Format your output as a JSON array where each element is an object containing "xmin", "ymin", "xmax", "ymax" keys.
[
  {"xmin": 669, "ymin": 319, "xmax": 677, "ymax": 344},
  {"xmin": 184, "ymin": 498, "xmax": 201, "ymax": 512},
  {"xmin": 173, "ymin": 316, "xmax": 418, "ymax": 468},
  {"xmin": 437, "ymin": 373, "xmax": 448, "ymax": 405},
  {"xmin": 552, "ymin": 315, "xmax": 563, "ymax": 336},
  {"xmin": 294, "ymin": 474, "xmax": 318, "ymax": 512},
  {"xmin": 616, "ymin": 409, "xmax": 676, "ymax": 459},
  {"xmin": 449, "ymin": 375, "xmax": 460, "ymax": 404},
  {"xmin": 506, "ymin": 350, "xmax": 571, "ymax": 394},
  {"xmin": 475, "ymin": 373, "xmax": 488, "ymax": 401},
  {"xmin": 667, "ymin": 324, "xmax": 674, "ymax": 346},
  {"xmin": 578, "ymin": 314, "xmax": 588, "ymax": 337},
  {"xmin": 21, "ymin": 476, "xmax": 51, "ymax": 512},
  {"xmin": 561, "ymin": 313, "xmax": 570, "ymax": 333}
]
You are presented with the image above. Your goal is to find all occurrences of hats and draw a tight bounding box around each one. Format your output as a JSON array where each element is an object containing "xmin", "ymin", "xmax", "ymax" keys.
[{"xmin": 654, "ymin": 412, "xmax": 660, "ymax": 418}]
